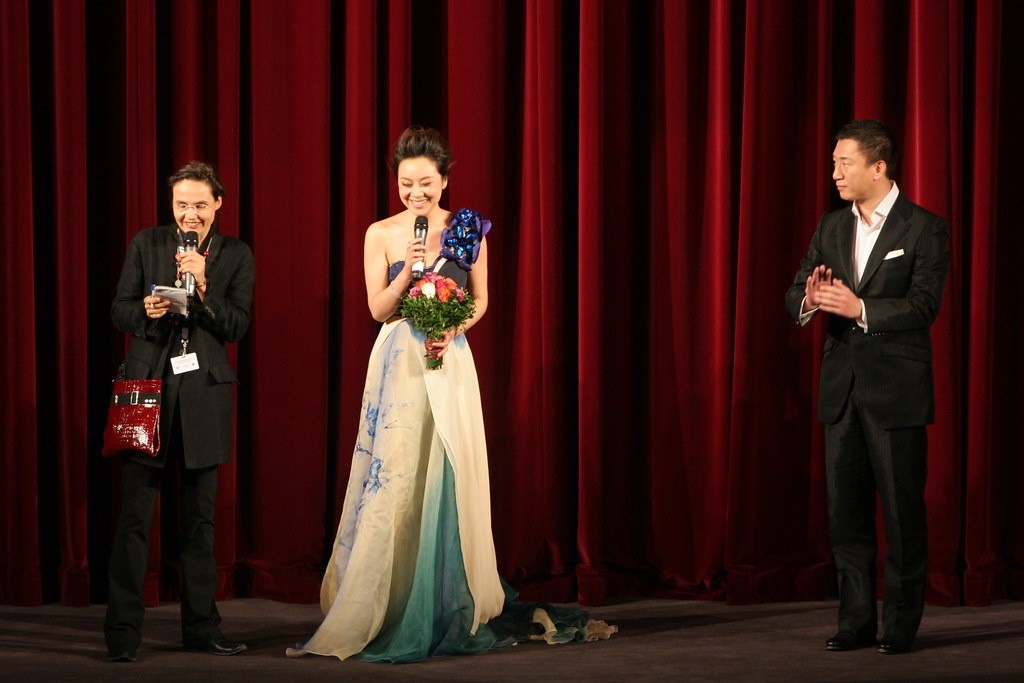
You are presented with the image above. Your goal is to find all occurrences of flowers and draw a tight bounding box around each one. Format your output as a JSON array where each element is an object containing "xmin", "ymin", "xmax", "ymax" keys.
[{"xmin": 392, "ymin": 271, "xmax": 477, "ymax": 371}]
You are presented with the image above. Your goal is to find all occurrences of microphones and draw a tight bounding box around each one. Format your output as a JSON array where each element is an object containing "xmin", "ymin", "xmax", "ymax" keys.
[
  {"xmin": 184, "ymin": 231, "xmax": 198, "ymax": 296},
  {"xmin": 411, "ymin": 214, "xmax": 427, "ymax": 282}
]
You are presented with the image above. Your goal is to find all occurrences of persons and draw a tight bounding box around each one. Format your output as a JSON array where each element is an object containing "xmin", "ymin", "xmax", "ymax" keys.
[
  {"xmin": 101, "ymin": 160, "xmax": 254, "ymax": 665},
  {"xmin": 284, "ymin": 126, "xmax": 618, "ymax": 661},
  {"xmin": 785, "ymin": 119, "xmax": 949, "ymax": 656}
]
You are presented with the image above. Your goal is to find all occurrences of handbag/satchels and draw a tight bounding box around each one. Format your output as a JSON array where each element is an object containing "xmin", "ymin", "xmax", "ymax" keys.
[{"xmin": 101, "ymin": 378, "xmax": 163, "ymax": 457}]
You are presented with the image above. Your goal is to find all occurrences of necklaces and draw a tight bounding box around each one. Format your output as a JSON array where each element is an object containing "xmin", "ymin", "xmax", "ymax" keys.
[{"xmin": 175, "ymin": 238, "xmax": 211, "ymax": 289}]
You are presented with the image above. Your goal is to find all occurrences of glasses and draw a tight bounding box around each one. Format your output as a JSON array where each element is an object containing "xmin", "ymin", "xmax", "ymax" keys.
[{"xmin": 172, "ymin": 198, "xmax": 216, "ymax": 215}]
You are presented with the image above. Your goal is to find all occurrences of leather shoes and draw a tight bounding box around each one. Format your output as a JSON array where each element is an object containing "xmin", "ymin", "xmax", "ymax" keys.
[
  {"xmin": 877, "ymin": 632, "xmax": 910, "ymax": 653},
  {"xmin": 106, "ymin": 639, "xmax": 137, "ymax": 661},
  {"xmin": 183, "ymin": 637, "xmax": 248, "ymax": 655},
  {"xmin": 826, "ymin": 627, "xmax": 876, "ymax": 649}
]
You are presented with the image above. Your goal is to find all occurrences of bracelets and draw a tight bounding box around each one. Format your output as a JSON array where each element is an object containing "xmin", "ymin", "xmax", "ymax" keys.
[
  {"xmin": 389, "ymin": 282, "xmax": 407, "ymax": 299},
  {"xmin": 196, "ymin": 279, "xmax": 207, "ymax": 288}
]
